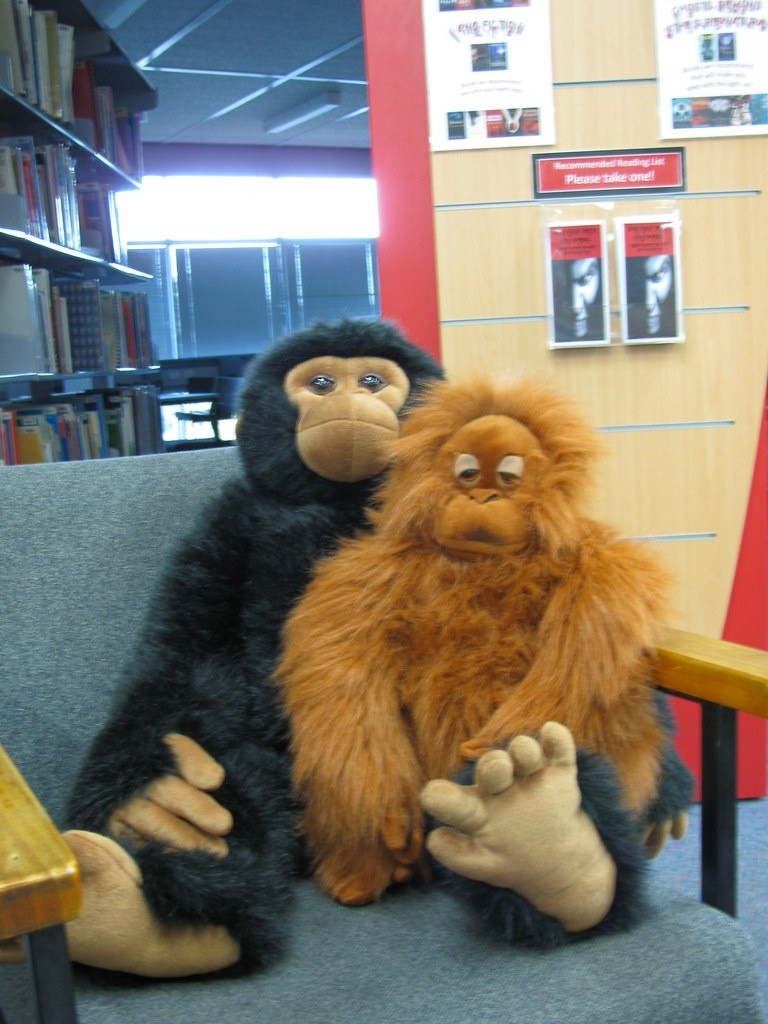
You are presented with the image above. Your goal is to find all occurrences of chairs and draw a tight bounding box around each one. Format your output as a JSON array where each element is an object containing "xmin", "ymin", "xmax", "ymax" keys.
[
  {"xmin": 177, "ymin": 377, "xmax": 243, "ymax": 438},
  {"xmin": 0, "ymin": 440, "xmax": 768, "ymax": 1024}
]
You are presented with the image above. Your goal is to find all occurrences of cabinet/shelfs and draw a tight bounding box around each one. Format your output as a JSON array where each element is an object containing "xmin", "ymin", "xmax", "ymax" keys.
[{"xmin": 0, "ymin": 0, "xmax": 158, "ymax": 462}]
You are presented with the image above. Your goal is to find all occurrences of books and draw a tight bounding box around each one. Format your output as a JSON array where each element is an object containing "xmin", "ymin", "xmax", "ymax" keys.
[
  {"xmin": 0, "ymin": 261, "xmax": 155, "ymax": 374},
  {"xmin": 0, "ymin": 134, "xmax": 128, "ymax": 267},
  {"xmin": 0, "ymin": 2, "xmax": 145, "ymax": 184},
  {"xmin": 0, "ymin": 383, "xmax": 163, "ymax": 464},
  {"xmin": 126, "ymin": 237, "xmax": 380, "ymax": 361}
]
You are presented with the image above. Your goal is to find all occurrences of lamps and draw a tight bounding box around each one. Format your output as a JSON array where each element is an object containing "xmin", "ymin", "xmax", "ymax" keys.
[{"xmin": 263, "ymin": 93, "xmax": 340, "ymax": 133}]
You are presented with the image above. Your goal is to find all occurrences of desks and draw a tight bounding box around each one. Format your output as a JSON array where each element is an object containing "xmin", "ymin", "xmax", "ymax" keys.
[{"xmin": 159, "ymin": 392, "xmax": 219, "ymax": 405}]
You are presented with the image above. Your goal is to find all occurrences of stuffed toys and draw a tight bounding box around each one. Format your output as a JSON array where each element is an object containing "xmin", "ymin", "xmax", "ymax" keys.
[
  {"xmin": 269, "ymin": 373, "xmax": 676, "ymax": 907},
  {"xmin": 59, "ymin": 316, "xmax": 697, "ymax": 986}
]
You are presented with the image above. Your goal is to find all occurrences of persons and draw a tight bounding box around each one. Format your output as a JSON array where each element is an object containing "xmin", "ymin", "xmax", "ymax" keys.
[
  {"xmin": 551, "ymin": 258, "xmax": 606, "ymax": 341},
  {"xmin": 625, "ymin": 253, "xmax": 676, "ymax": 340}
]
